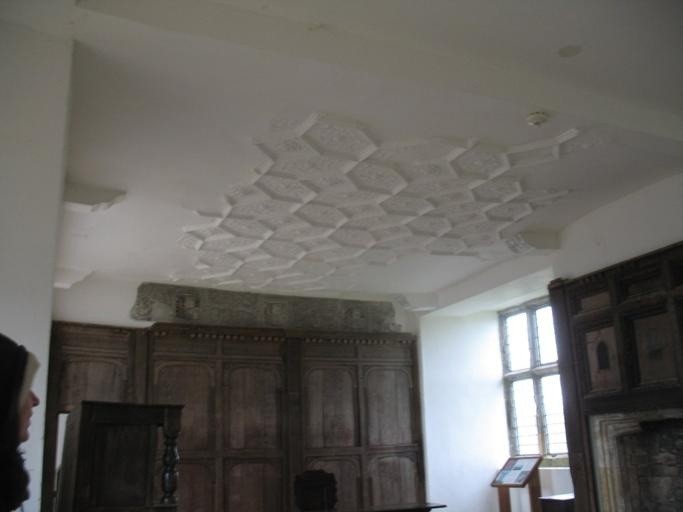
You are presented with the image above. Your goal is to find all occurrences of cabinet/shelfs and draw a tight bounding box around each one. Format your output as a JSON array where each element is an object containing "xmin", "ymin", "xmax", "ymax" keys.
[
  {"xmin": 293, "ymin": 330, "xmax": 426, "ymax": 512},
  {"xmin": 48, "ymin": 320, "xmax": 147, "ymax": 412},
  {"xmin": 536, "ymin": 491, "xmax": 575, "ymax": 511},
  {"xmin": 149, "ymin": 323, "xmax": 293, "ymax": 512},
  {"xmin": 60, "ymin": 399, "xmax": 185, "ymax": 511}
]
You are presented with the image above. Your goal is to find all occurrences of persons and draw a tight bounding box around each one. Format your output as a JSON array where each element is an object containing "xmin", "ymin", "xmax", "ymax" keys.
[{"xmin": 1, "ymin": 334, "xmax": 40, "ymax": 511}]
